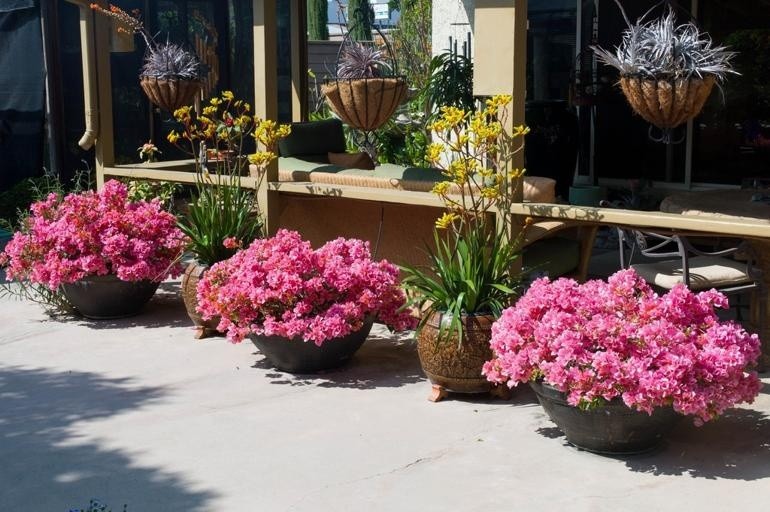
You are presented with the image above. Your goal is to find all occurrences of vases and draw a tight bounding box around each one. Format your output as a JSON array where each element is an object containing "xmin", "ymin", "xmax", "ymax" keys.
[
  {"xmin": 62, "ymin": 275, "xmax": 161, "ymax": 320},
  {"xmin": 249, "ymin": 311, "xmax": 375, "ymax": 375},
  {"xmin": 183, "ymin": 261, "xmax": 249, "ymax": 341},
  {"xmin": 530, "ymin": 380, "xmax": 686, "ymax": 456},
  {"xmin": 415, "ymin": 307, "xmax": 521, "ymax": 403}
]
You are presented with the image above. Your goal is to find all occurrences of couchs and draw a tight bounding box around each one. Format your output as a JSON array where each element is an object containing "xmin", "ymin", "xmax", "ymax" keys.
[{"xmin": 249, "ymin": 119, "xmax": 600, "ymax": 306}]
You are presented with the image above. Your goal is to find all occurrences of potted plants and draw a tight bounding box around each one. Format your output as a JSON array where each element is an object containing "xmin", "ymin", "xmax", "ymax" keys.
[
  {"xmin": 590, "ymin": 0, "xmax": 738, "ymax": 145},
  {"xmin": 321, "ymin": 20, "xmax": 408, "ymax": 131},
  {"xmin": 142, "ymin": 35, "xmax": 209, "ymax": 116}
]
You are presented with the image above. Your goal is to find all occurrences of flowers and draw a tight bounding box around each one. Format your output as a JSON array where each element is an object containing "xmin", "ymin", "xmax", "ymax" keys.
[
  {"xmin": 89, "ymin": 2, "xmax": 143, "ymax": 35},
  {"xmin": 391, "ymin": 93, "xmax": 537, "ymax": 350},
  {"xmin": 198, "ymin": 229, "xmax": 418, "ymax": 346},
  {"xmin": 168, "ymin": 90, "xmax": 291, "ymax": 268},
  {"xmin": 0, "ymin": 179, "xmax": 191, "ymax": 290},
  {"xmin": 482, "ymin": 267, "xmax": 762, "ymax": 431},
  {"xmin": 620, "ymin": 178, "xmax": 662, "ymax": 209}
]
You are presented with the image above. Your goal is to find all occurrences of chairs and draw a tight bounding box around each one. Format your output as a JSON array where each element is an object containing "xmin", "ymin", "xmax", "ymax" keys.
[
  {"xmin": 602, "ymin": 201, "xmax": 760, "ymax": 336},
  {"xmin": 524, "ymin": 100, "xmax": 578, "ymax": 204}
]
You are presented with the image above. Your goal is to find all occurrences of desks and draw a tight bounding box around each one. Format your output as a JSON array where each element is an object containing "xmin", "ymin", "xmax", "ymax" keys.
[{"xmin": 658, "ymin": 188, "xmax": 770, "ymax": 370}]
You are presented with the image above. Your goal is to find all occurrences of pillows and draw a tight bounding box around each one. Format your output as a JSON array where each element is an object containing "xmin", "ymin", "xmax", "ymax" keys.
[
  {"xmin": 327, "ymin": 151, "xmax": 376, "ymax": 169},
  {"xmin": 276, "ymin": 117, "xmax": 347, "ymax": 156}
]
